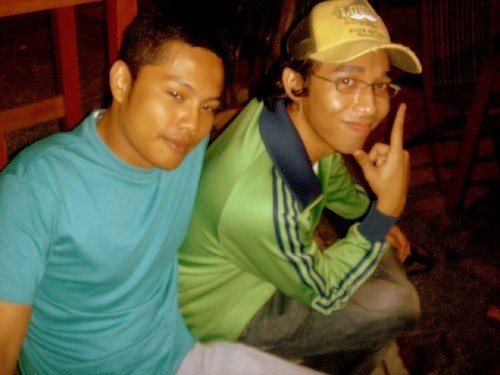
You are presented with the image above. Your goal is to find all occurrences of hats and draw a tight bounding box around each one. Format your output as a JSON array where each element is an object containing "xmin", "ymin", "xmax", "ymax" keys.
[{"xmin": 287, "ymin": 0, "xmax": 423, "ymax": 74}]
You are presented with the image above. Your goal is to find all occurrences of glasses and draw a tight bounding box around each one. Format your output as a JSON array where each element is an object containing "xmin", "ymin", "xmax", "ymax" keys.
[{"xmin": 307, "ymin": 70, "xmax": 402, "ymax": 100}]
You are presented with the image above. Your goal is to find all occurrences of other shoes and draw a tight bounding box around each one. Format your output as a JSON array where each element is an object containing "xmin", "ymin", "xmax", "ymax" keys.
[{"xmin": 404, "ymin": 239, "xmax": 436, "ymax": 277}]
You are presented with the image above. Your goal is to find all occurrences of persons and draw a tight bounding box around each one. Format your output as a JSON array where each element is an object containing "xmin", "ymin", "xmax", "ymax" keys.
[
  {"xmin": 0, "ymin": 6, "xmax": 326, "ymax": 375},
  {"xmin": 176, "ymin": 1, "xmax": 425, "ymax": 375}
]
